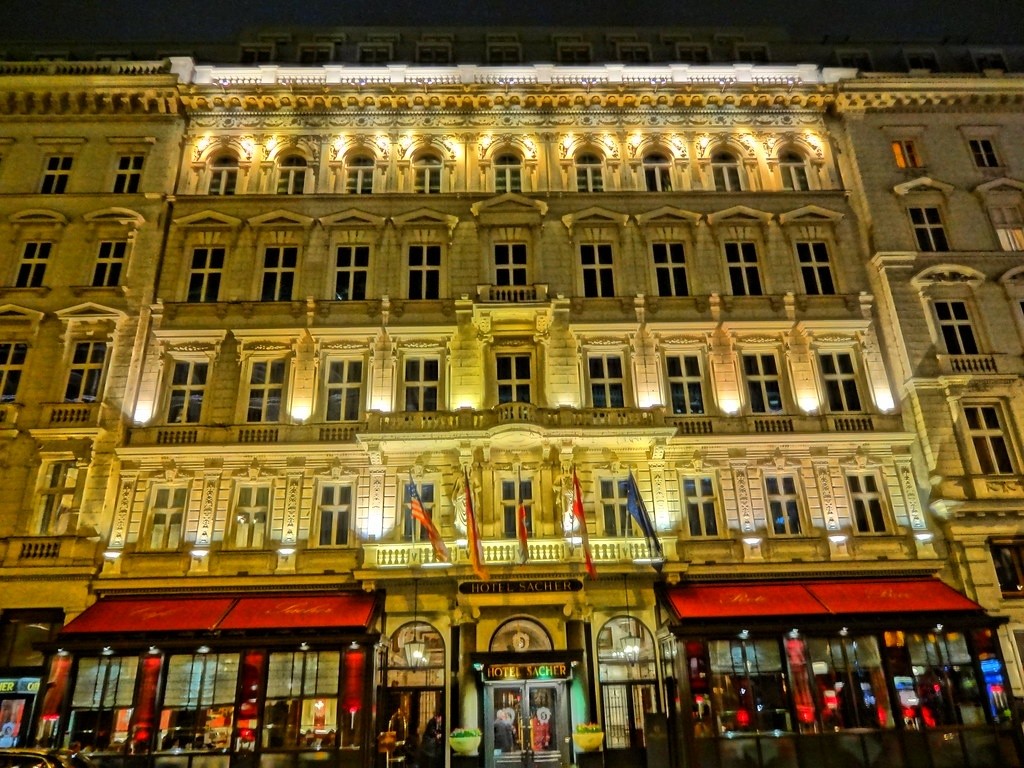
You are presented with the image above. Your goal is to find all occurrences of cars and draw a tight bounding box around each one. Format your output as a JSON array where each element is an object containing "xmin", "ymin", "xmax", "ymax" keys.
[{"xmin": 0, "ymin": 748, "xmax": 75, "ymax": 768}]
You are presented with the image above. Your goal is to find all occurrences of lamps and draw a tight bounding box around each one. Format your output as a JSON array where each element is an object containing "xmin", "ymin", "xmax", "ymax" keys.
[
  {"xmin": 404, "ymin": 580, "xmax": 426, "ymax": 673},
  {"xmin": 620, "ymin": 577, "xmax": 641, "ymax": 666}
]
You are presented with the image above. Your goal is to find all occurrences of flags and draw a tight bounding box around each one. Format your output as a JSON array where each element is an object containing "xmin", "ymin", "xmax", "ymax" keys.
[
  {"xmin": 465, "ymin": 466, "xmax": 491, "ymax": 581},
  {"xmin": 573, "ymin": 468, "xmax": 597, "ymax": 579},
  {"xmin": 518, "ymin": 499, "xmax": 529, "ymax": 562},
  {"xmin": 408, "ymin": 462, "xmax": 450, "ymax": 561},
  {"xmin": 628, "ymin": 470, "xmax": 659, "ymax": 552}
]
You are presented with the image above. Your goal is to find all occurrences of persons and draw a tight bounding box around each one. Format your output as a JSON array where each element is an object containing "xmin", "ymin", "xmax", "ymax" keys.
[
  {"xmin": 0, "ymin": 722, "xmax": 18, "ymax": 748},
  {"xmin": 421, "ymin": 714, "xmax": 442, "ymax": 758},
  {"xmin": 532, "ymin": 711, "xmax": 550, "ymax": 752},
  {"xmin": 69, "ymin": 742, "xmax": 88, "ymax": 768},
  {"xmin": 494, "ymin": 708, "xmax": 517, "ymax": 754}
]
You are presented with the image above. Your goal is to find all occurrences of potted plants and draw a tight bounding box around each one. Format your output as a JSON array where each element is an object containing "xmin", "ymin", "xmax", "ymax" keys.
[{"xmin": 448, "ymin": 728, "xmax": 482, "ymax": 738}]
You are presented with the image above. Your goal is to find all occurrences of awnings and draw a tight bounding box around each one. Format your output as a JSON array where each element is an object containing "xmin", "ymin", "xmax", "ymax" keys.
[
  {"xmin": 59, "ymin": 592, "xmax": 378, "ymax": 633},
  {"xmin": 665, "ymin": 572, "xmax": 989, "ymax": 630}
]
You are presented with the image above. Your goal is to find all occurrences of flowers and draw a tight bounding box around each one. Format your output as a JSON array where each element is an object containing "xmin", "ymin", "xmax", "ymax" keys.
[{"xmin": 575, "ymin": 722, "xmax": 601, "ymax": 734}]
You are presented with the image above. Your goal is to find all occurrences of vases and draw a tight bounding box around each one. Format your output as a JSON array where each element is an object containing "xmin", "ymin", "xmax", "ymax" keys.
[
  {"xmin": 572, "ymin": 732, "xmax": 605, "ymax": 752},
  {"xmin": 448, "ymin": 736, "xmax": 481, "ymax": 756}
]
